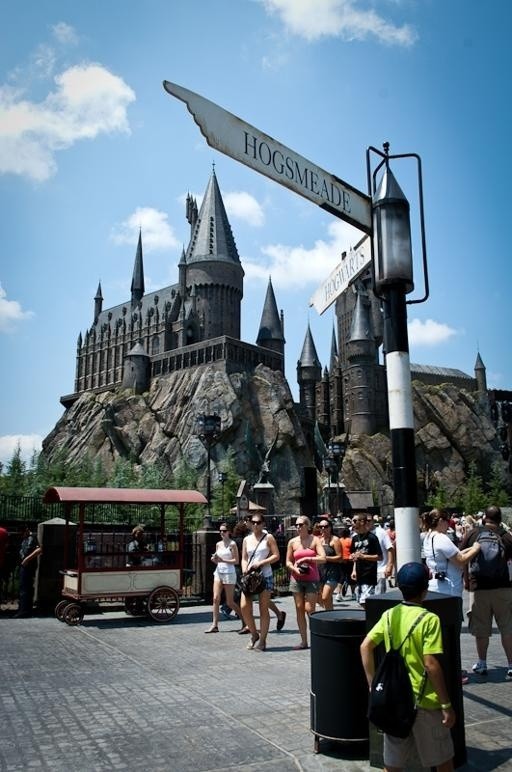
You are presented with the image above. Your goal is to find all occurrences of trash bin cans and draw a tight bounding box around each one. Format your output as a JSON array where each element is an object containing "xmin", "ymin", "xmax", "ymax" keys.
[{"xmin": 309, "ymin": 609, "xmax": 370, "ymax": 761}]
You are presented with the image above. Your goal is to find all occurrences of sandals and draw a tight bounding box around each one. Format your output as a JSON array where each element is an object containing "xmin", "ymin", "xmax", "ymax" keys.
[{"xmin": 204, "ymin": 625, "xmax": 220, "ymax": 632}]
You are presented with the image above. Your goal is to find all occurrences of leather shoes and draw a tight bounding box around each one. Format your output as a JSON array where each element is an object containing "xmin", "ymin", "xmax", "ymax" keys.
[
  {"xmin": 256, "ymin": 645, "xmax": 266, "ymax": 652},
  {"xmin": 277, "ymin": 611, "xmax": 286, "ymax": 630},
  {"xmin": 238, "ymin": 627, "xmax": 249, "ymax": 634},
  {"xmin": 246, "ymin": 630, "xmax": 259, "ymax": 650}
]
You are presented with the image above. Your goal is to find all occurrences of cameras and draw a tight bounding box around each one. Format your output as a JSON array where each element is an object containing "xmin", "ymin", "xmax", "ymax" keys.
[
  {"xmin": 299, "ymin": 566, "xmax": 309, "ymax": 574},
  {"xmin": 435, "ymin": 571, "xmax": 446, "ymax": 580}
]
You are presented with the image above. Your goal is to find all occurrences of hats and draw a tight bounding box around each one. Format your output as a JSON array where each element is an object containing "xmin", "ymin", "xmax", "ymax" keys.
[
  {"xmin": 397, "ymin": 562, "xmax": 430, "ymax": 592},
  {"xmin": 131, "ymin": 525, "xmax": 145, "ymax": 536}
]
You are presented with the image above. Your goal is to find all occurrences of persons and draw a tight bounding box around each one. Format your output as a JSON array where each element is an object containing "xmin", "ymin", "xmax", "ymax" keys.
[
  {"xmin": 13, "ymin": 524, "xmax": 42, "ymax": 618},
  {"xmin": 127, "ymin": 526, "xmax": 176, "ymax": 569},
  {"xmin": 359, "ymin": 562, "xmax": 456, "ymax": 771},
  {"xmin": 206, "ymin": 505, "xmax": 511, "ymax": 680}
]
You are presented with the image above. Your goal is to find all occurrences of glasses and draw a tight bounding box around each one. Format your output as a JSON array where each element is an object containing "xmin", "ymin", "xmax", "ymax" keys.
[
  {"xmin": 251, "ymin": 521, "xmax": 261, "ymax": 525},
  {"xmin": 353, "ymin": 519, "xmax": 357, "ymax": 524},
  {"xmin": 220, "ymin": 530, "xmax": 229, "ymax": 533},
  {"xmin": 295, "ymin": 524, "xmax": 304, "ymax": 527},
  {"xmin": 318, "ymin": 524, "xmax": 328, "ymax": 529}
]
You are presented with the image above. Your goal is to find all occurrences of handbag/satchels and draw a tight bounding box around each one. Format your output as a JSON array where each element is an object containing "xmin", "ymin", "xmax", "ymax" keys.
[{"xmin": 240, "ymin": 566, "xmax": 266, "ymax": 595}]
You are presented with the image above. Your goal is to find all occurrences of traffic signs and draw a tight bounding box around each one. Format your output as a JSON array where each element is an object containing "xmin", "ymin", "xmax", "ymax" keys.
[
  {"xmin": 305, "ymin": 232, "xmax": 372, "ymax": 314},
  {"xmin": 166, "ymin": 79, "xmax": 374, "ymax": 237}
]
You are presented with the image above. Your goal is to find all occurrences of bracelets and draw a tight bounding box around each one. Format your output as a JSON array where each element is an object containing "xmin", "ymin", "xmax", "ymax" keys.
[{"xmin": 441, "ymin": 702, "xmax": 452, "ymax": 709}]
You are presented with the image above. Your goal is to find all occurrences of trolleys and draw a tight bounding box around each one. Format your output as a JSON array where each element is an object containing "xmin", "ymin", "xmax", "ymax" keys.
[{"xmin": 41, "ymin": 485, "xmax": 208, "ymax": 626}]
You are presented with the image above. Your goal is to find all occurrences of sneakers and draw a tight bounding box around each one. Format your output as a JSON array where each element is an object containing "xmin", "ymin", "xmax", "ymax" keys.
[
  {"xmin": 220, "ymin": 607, "xmax": 231, "ymax": 620},
  {"xmin": 292, "ymin": 645, "xmax": 308, "ymax": 649},
  {"xmin": 472, "ymin": 662, "xmax": 487, "ymax": 675},
  {"xmin": 335, "ymin": 584, "xmax": 361, "ymax": 604},
  {"xmin": 504, "ymin": 666, "xmax": 512, "ymax": 679}
]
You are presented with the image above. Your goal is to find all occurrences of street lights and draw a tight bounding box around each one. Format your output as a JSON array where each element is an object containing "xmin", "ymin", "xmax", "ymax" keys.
[
  {"xmin": 365, "ymin": 129, "xmax": 427, "ymax": 595},
  {"xmin": 196, "ymin": 411, "xmax": 224, "ymax": 530},
  {"xmin": 325, "ymin": 451, "xmax": 341, "ymax": 524},
  {"xmin": 328, "ymin": 439, "xmax": 347, "ymax": 519}
]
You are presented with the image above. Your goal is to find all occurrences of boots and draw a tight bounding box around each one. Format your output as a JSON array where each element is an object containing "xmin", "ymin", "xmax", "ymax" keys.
[{"xmin": 9, "ymin": 593, "xmax": 33, "ymax": 618}]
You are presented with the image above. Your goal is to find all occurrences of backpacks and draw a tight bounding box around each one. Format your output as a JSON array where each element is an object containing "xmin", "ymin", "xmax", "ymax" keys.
[
  {"xmin": 366, "ymin": 609, "xmax": 430, "ymax": 739},
  {"xmin": 468, "ymin": 525, "xmax": 510, "ymax": 591}
]
